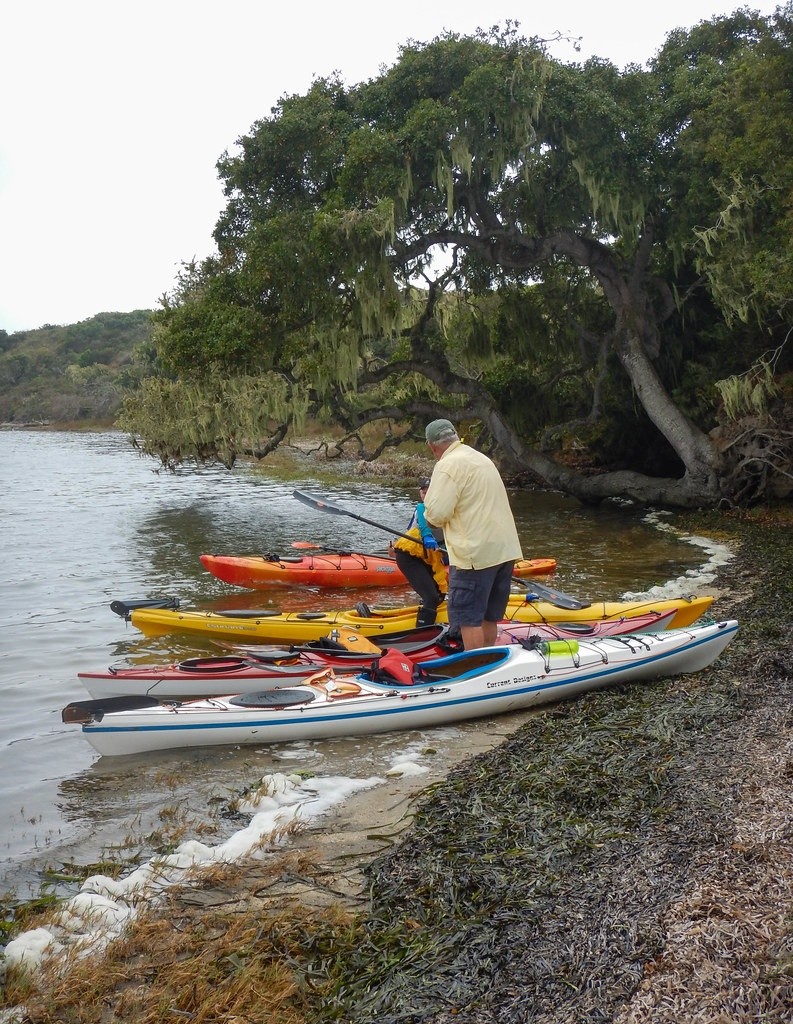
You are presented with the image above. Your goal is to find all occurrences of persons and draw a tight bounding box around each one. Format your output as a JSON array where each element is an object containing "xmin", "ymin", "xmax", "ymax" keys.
[
  {"xmin": 394, "ymin": 502, "xmax": 449, "ymax": 628},
  {"xmin": 406, "ymin": 479, "xmax": 431, "ymax": 530},
  {"xmin": 423, "ymin": 418, "xmax": 523, "ymax": 651}
]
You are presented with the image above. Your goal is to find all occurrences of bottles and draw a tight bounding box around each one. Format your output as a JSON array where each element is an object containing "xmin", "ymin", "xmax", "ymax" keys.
[{"xmin": 539, "ymin": 639, "xmax": 579, "ymax": 655}]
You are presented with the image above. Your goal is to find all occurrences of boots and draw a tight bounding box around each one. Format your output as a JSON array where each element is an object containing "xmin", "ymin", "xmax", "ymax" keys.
[{"xmin": 415, "ymin": 607, "xmax": 437, "ymax": 627}]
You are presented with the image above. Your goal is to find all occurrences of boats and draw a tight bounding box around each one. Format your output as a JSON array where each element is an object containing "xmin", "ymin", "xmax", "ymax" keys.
[
  {"xmin": 109, "ymin": 594, "xmax": 716, "ymax": 645},
  {"xmin": 193, "ymin": 550, "xmax": 559, "ymax": 590},
  {"xmin": 75, "ymin": 604, "xmax": 681, "ymax": 700},
  {"xmin": 61, "ymin": 617, "xmax": 744, "ymax": 758}
]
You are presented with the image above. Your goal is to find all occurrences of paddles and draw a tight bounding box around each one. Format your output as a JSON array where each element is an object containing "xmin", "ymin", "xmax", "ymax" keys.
[
  {"xmin": 293, "ymin": 541, "xmax": 397, "ymax": 562},
  {"xmin": 208, "ymin": 638, "xmax": 371, "ymax": 656},
  {"xmin": 293, "ymin": 488, "xmax": 588, "ymax": 610}
]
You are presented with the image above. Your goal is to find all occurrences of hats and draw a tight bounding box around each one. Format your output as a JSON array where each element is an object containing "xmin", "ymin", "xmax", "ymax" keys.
[
  {"xmin": 421, "ymin": 478, "xmax": 431, "ymax": 490},
  {"xmin": 425, "ymin": 419, "xmax": 457, "ymax": 444}
]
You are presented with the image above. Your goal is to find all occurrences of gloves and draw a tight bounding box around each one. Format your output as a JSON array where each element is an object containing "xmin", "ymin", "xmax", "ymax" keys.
[{"xmin": 424, "ymin": 535, "xmax": 438, "ymax": 551}]
